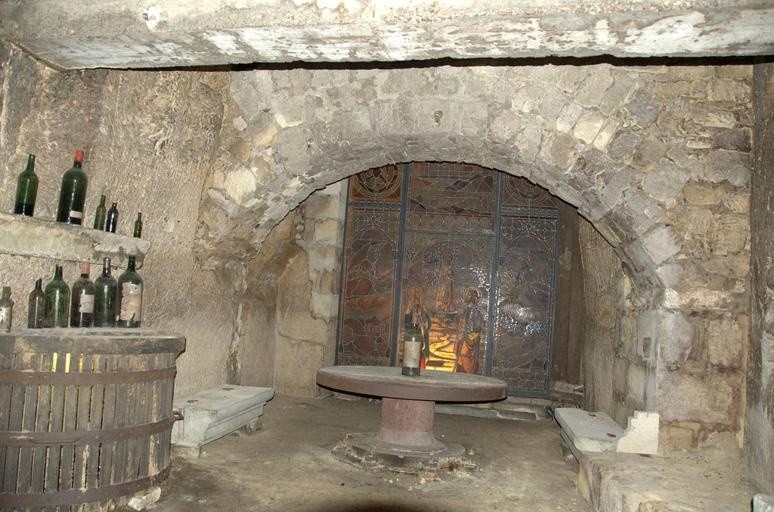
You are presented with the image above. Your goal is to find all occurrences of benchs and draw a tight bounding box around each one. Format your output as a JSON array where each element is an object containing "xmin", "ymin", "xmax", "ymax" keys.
[
  {"xmin": 174, "ymin": 385, "xmax": 274, "ymax": 459},
  {"xmin": 554, "ymin": 408, "xmax": 625, "ymax": 478}
]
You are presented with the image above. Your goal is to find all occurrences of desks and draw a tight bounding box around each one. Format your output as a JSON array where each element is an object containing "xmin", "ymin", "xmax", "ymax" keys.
[{"xmin": 316, "ymin": 364, "xmax": 508, "ymax": 474}]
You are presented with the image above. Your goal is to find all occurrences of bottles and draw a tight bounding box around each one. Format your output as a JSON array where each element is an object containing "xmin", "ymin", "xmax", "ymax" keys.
[
  {"xmin": 26, "ymin": 255, "xmax": 143, "ymax": 327},
  {"xmin": 0, "ymin": 287, "xmax": 12, "ymax": 332},
  {"xmin": 401, "ymin": 314, "xmax": 423, "ymax": 377},
  {"xmin": 132, "ymin": 212, "xmax": 142, "ymax": 238},
  {"xmin": 105, "ymin": 202, "xmax": 117, "ymax": 233},
  {"xmin": 93, "ymin": 193, "xmax": 106, "ymax": 230},
  {"xmin": 12, "ymin": 153, "xmax": 39, "ymax": 217},
  {"xmin": 55, "ymin": 150, "xmax": 87, "ymax": 226}
]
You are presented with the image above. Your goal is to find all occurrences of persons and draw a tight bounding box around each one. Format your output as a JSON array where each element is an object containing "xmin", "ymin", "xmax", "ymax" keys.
[
  {"xmin": 456, "ymin": 288, "xmax": 484, "ymax": 373},
  {"xmin": 405, "ymin": 297, "xmax": 431, "ymax": 368}
]
